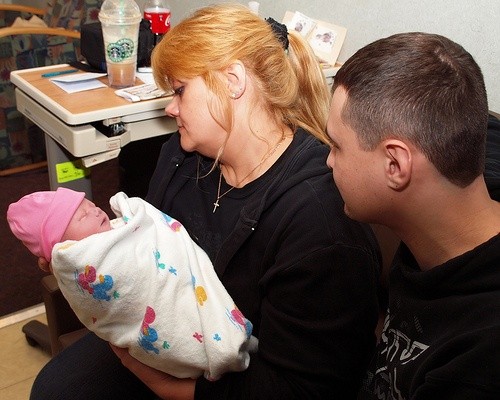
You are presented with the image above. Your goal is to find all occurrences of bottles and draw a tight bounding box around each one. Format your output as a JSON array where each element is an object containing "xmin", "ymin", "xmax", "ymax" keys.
[{"xmin": 145, "ymin": 0, "xmax": 171, "ymax": 60}]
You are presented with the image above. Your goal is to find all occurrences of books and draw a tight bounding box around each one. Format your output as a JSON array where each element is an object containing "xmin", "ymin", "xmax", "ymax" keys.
[{"xmin": 277, "ymin": 11, "xmax": 348, "ymax": 67}]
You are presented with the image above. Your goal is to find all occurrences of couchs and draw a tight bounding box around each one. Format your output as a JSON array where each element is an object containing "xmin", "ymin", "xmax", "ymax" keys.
[{"xmin": 0, "ymin": 0, "xmax": 103, "ymax": 176}]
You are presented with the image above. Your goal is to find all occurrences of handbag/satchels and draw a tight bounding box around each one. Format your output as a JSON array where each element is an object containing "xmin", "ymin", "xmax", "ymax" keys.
[{"xmin": 66, "ymin": 17, "xmax": 155, "ymax": 74}]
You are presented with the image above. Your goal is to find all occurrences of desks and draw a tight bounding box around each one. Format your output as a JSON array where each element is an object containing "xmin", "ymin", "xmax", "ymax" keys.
[{"xmin": 9, "ymin": 61, "xmax": 344, "ymax": 359}]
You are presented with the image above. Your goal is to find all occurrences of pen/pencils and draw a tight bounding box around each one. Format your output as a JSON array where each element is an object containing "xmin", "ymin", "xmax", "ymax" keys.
[{"xmin": 42, "ymin": 69, "xmax": 78, "ymax": 79}]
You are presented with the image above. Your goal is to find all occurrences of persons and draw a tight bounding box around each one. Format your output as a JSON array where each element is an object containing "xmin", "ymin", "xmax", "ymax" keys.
[
  {"xmin": 7, "ymin": 186, "xmax": 258, "ymax": 378},
  {"xmin": 30, "ymin": 4, "xmax": 384, "ymax": 400},
  {"xmin": 327, "ymin": 33, "xmax": 500, "ymax": 400}
]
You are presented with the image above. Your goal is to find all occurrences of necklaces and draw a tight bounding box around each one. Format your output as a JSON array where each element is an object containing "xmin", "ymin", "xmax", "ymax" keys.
[{"xmin": 213, "ymin": 125, "xmax": 287, "ymax": 212}]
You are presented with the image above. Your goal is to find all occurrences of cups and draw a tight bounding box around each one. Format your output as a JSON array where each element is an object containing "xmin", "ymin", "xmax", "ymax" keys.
[{"xmin": 98, "ymin": 0, "xmax": 142, "ymax": 87}]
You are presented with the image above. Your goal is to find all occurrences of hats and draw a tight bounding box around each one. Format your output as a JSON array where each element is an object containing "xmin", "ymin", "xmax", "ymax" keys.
[{"xmin": 7, "ymin": 187, "xmax": 86, "ymax": 263}]
